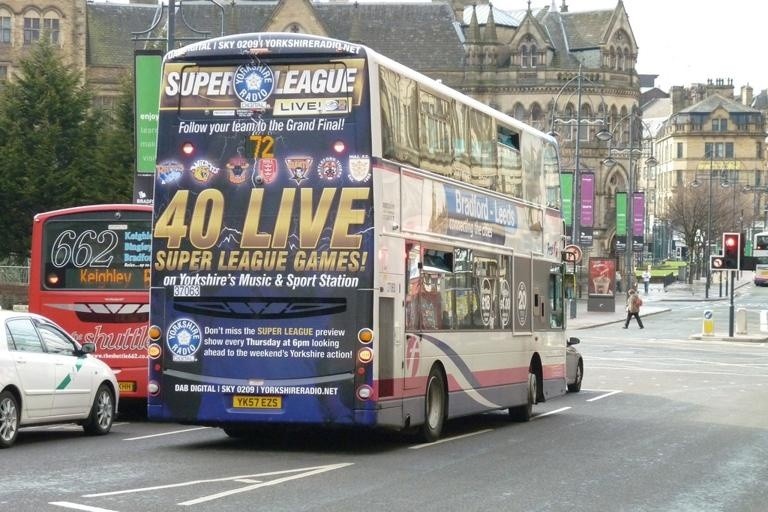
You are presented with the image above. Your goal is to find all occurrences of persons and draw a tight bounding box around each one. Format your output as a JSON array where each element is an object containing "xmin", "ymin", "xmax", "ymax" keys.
[
  {"xmin": 623, "ymin": 289, "xmax": 644, "ymax": 329},
  {"xmin": 642, "ymin": 268, "xmax": 651, "ymax": 292}
]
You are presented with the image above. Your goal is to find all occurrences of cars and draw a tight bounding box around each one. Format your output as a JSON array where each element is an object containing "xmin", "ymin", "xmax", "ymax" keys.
[
  {"xmin": 566, "ymin": 335, "xmax": 584, "ymax": 391},
  {"xmin": 0, "ymin": 309, "xmax": 120, "ymax": 449}
]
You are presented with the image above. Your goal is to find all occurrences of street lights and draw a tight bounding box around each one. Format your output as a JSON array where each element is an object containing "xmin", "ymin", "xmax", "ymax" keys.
[
  {"xmin": 545, "ymin": 58, "xmax": 614, "ymax": 246},
  {"xmin": 595, "ymin": 102, "xmax": 659, "ymax": 308},
  {"xmin": 720, "ymin": 153, "xmax": 752, "ymax": 231},
  {"xmin": 690, "ymin": 141, "xmax": 730, "ymax": 288}
]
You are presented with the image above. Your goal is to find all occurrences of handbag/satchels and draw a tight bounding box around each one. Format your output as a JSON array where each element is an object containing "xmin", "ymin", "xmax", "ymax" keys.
[{"xmin": 634, "ymin": 298, "xmax": 642, "ymax": 306}]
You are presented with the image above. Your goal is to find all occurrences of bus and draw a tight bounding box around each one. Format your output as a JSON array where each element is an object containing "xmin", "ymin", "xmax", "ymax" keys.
[
  {"xmin": 753, "ymin": 231, "xmax": 768, "ymax": 287},
  {"xmin": 146, "ymin": 30, "xmax": 569, "ymax": 444},
  {"xmin": 27, "ymin": 203, "xmax": 154, "ymax": 401}
]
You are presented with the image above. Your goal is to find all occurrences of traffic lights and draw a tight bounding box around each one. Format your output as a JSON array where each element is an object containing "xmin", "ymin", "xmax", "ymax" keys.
[{"xmin": 709, "ymin": 232, "xmax": 741, "ymax": 272}]
